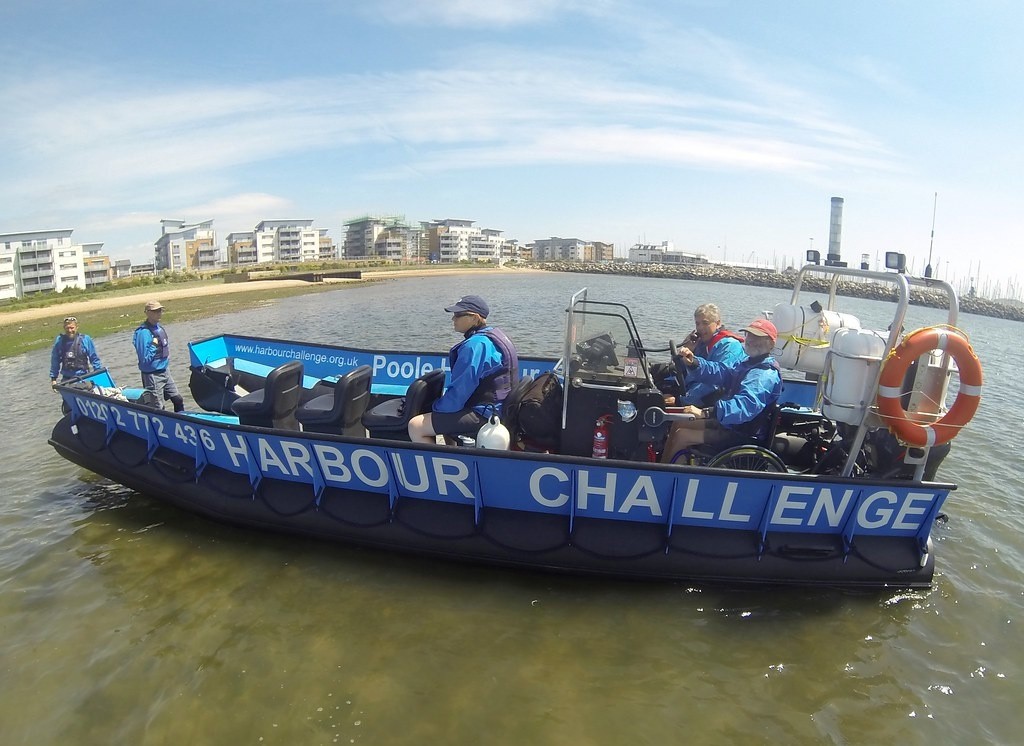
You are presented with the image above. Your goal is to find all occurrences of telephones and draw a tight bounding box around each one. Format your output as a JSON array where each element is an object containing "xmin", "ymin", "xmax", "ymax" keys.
[{"xmin": 690, "ymin": 331, "xmax": 701, "ymax": 342}]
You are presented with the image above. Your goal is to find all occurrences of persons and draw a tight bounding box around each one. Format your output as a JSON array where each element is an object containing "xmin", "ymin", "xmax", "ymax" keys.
[
  {"xmin": 665, "ymin": 303, "xmax": 749, "ymax": 406},
  {"xmin": 50, "ymin": 315, "xmax": 102, "ymax": 393},
  {"xmin": 132, "ymin": 301, "xmax": 184, "ymax": 412},
  {"xmin": 408, "ymin": 296, "xmax": 519, "ymax": 445},
  {"xmin": 661, "ymin": 319, "xmax": 784, "ymax": 464}
]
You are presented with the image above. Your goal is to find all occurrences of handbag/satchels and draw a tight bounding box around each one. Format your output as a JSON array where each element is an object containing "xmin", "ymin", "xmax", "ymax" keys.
[{"xmin": 517, "ymin": 370, "xmax": 563, "ymax": 441}]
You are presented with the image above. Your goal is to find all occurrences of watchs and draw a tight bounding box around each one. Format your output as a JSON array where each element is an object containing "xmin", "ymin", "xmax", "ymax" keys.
[{"xmin": 703, "ymin": 408, "xmax": 709, "ymax": 419}]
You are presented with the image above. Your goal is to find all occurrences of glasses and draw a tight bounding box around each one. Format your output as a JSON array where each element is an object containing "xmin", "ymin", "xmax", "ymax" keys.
[
  {"xmin": 454, "ymin": 312, "xmax": 476, "ymax": 318},
  {"xmin": 64, "ymin": 317, "xmax": 76, "ymax": 322}
]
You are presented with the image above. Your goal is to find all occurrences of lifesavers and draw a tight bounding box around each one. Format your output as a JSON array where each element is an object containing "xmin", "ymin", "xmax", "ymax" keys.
[{"xmin": 877, "ymin": 323, "xmax": 982, "ymax": 449}]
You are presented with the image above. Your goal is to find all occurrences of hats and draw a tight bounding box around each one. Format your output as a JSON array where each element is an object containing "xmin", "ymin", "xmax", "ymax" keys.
[
  {"xmin": 738, "ymin": 318, "xmax": 778, "ymax": 346},
  {"xmin": 444, "ymin": 294, "xmax": 489, "ymax": 319},
  {"xmin": 144, "ymin": 301, "xmax": 165, "ymax": 313}
]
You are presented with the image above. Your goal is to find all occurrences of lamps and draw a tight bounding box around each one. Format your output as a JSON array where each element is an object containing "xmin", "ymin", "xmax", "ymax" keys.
[
  {"xmin": 886, "ymin": 252, "xmax": 906, "ymax": 274},
  {"xmin": 807, "ymin": 250, "xmax": 820, "ymax": 265}
]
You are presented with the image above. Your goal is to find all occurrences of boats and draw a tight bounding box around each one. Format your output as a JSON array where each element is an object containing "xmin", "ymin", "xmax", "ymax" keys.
[{"xmin": 46, "ymin": 263, "xmax": 960, "ymax": 590}]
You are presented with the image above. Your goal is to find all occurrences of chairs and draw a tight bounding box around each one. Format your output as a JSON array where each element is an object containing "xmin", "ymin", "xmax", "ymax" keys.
[
  {"xmin": 294, "ymin": 365, "xmax": 373, "ymax": 438},
  {"xmin": 231, "ymin": 361, "xmax": 304, "ymax": 430},
  {"xmin": 361, "ymin": 368, "xmax": 446, "ymax": 441},
  {"xmin": 446, "ymin": 375, "xmax": 533, "ymax": 449}
]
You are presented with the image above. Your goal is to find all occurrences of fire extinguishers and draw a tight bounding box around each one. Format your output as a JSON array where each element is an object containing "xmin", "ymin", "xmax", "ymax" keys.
[{"xmin": 591, "ymin": 414, "xmax": 615, "ymax": 458}]
